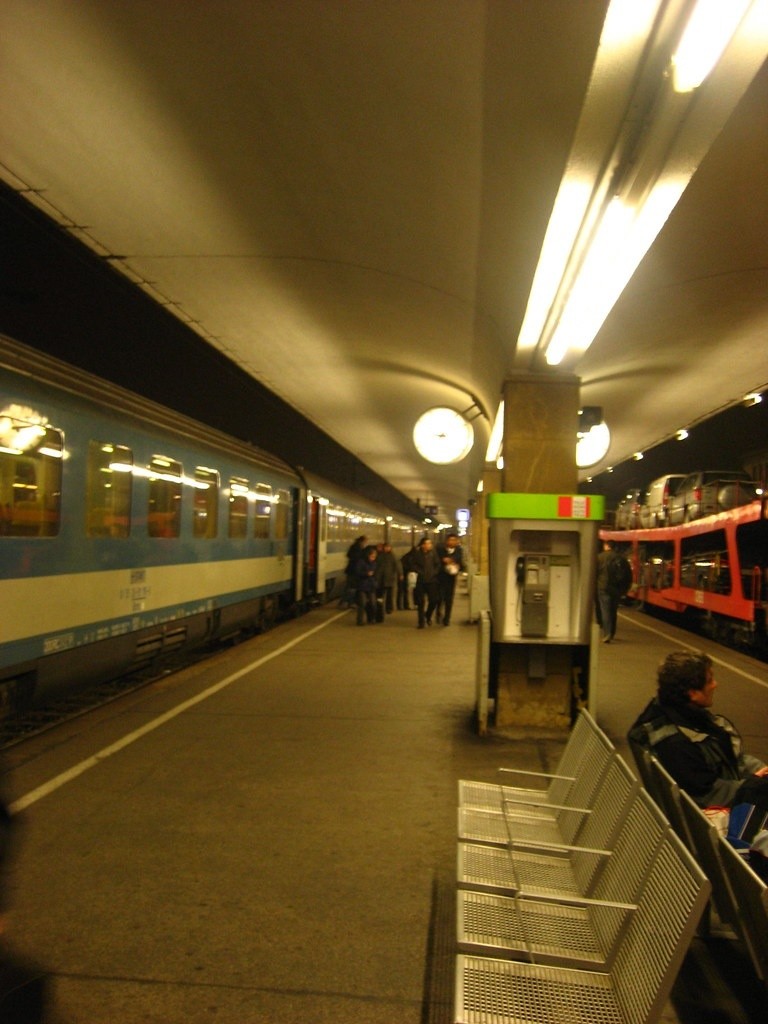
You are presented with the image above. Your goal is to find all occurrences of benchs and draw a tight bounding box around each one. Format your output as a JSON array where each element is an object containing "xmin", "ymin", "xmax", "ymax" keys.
[
  {"xmin": 632, "ymin": 740, "xmax": 768, "ymax": 992},
  {"xmin": 453, "ymin": 707, "xmax": 713, "ymax": 1024}
]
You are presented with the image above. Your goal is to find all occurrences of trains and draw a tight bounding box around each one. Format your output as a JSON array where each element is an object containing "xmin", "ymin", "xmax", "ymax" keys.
[
  {"xmin": 597, "ymin": 498, "xmax": 768, "ymax": 659},
  {"xmin": 0, "ymin": 332, "xmax": 434, "ymax": 722}
]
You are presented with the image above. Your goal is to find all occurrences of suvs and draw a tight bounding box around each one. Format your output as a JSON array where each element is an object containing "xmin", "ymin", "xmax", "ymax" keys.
[{"xmin": 666, "ymin": 471, "xmax": 756, "ymax": 526}]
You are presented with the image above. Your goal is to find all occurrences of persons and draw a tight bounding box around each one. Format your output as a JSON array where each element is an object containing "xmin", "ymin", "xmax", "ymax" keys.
[
  {"xmin": 337, "ymin": 533, "xmax": 466, "ymax": 629},
  {"xmin": 628, "ymin": 649, "xmax": 768, "ymax": 812},
  {"xmin": 594, "ymin": 539, "xmax": 621, "ymax": 643}
]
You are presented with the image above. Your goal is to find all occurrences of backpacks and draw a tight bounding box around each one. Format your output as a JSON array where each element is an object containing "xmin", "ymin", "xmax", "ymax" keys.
[{"xmin": 606, "ymin": 555, "xmax": 633, "ymax": 598}]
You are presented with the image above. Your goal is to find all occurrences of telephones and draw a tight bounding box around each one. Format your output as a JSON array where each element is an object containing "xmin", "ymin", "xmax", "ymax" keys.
[{"xmin": 517, "ymin": 553, "xmax": 551, "ymax": 590}]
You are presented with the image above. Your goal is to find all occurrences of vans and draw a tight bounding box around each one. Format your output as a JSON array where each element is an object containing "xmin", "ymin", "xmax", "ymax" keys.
[
  {"xmin": 638, "ymin": 474, "xmax": 687, "ymax": 529},
  {"xmin": 612, "ymin": 489, "xmax": 639, "ymax": 530}
]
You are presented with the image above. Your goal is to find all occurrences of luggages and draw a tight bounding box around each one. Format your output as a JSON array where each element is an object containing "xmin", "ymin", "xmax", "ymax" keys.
[{"xmin": 364, "ymin": 600, "xmax": 384, "ymax": 623}]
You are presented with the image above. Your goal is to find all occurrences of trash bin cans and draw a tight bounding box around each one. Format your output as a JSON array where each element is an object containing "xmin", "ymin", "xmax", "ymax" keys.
[{"xmin": 457, "ymin": 570, "xmax": 469, "ymax": 594}]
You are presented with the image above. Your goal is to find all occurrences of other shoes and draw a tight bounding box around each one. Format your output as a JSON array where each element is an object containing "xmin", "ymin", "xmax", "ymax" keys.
[
  {"xmin": 424, "ymin": 612, "xmax": 433, "ymax": 626},
  {"xmin": 417, "ymin": 623, "xmax": 425, "ymax": 628},
  {"xmin": 367, "ymin": 620, "xmax": 377, "ymax": 624},
  {"xmin": 435, "ymin": 610, "xmax": 441, "ymax": 616},
  {"xmin": 404, "ymin": 606, "xmax": 412, "ymax": 610},
  {"xmin": 602, "ymin": 635, "xmax": 611, "ymax": 643},
  {"xmin": 356, "ymin": 622, "xmax": 365, "ymax": 626},
  {"xmin": 442, "ymin": 619, "xmax": 449, "ymax": 626},
  {"xmin": 396, "ymin": 603, "xmax": 402, "ymax": 611}
]
